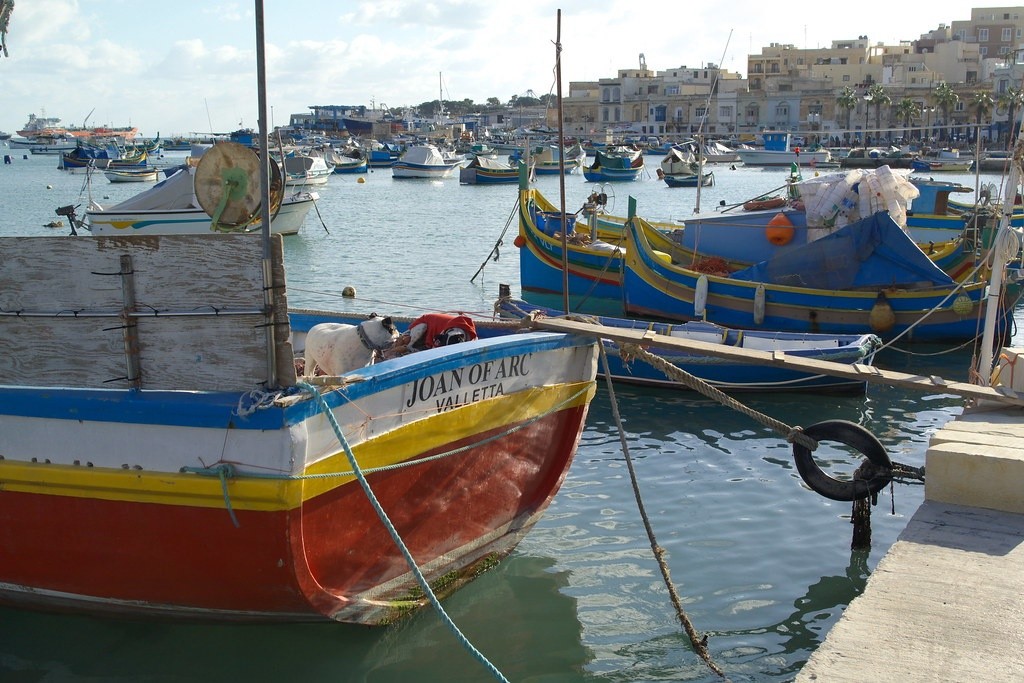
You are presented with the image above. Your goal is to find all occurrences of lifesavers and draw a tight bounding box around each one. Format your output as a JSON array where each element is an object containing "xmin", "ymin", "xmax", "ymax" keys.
[
  {"xmin": 743, "ymin": 196, "xmax": 786, "ymax": 210},
  {"xmin": 793, "ymin": 419, "xmax": 893, "ymax": 502}
]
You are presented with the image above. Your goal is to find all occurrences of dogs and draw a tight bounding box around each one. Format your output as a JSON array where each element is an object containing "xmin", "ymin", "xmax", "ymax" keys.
[
  {"xmin": 303, "ymin": 312, "xmax": 400, "ymax": 379},
  {"xmin": 403, "ymin": 313, "xmax": 478, "ymax": 354}
]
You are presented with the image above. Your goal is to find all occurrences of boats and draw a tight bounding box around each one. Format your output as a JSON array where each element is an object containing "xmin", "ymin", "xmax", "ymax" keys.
[
  {"xmin": 0, "ymin": 0, "xmax": 603, "ymax": 625},
  {"xmin": 494, "ymin": 284, "xmax": 881, "ymax": 390},
  {"xmin": 55, "ymin": 157, "xmax": 318, "ymax": 235},
  {"xmin": 519, "ymin": 9, "xmax": 1024, "ymax": 347},
  {"xmin": 0, "ymin": 72, "xmax": 1024, "ymax": 185},
  {"xmin": 664, "ymin": 172, "xmax": 712, "ymax": 189}
]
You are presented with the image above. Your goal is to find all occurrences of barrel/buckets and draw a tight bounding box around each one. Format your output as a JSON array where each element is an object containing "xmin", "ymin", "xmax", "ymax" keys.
[{"xmin": 806, "ymin": 164, "xmax": 920, "ymax": 225}]
